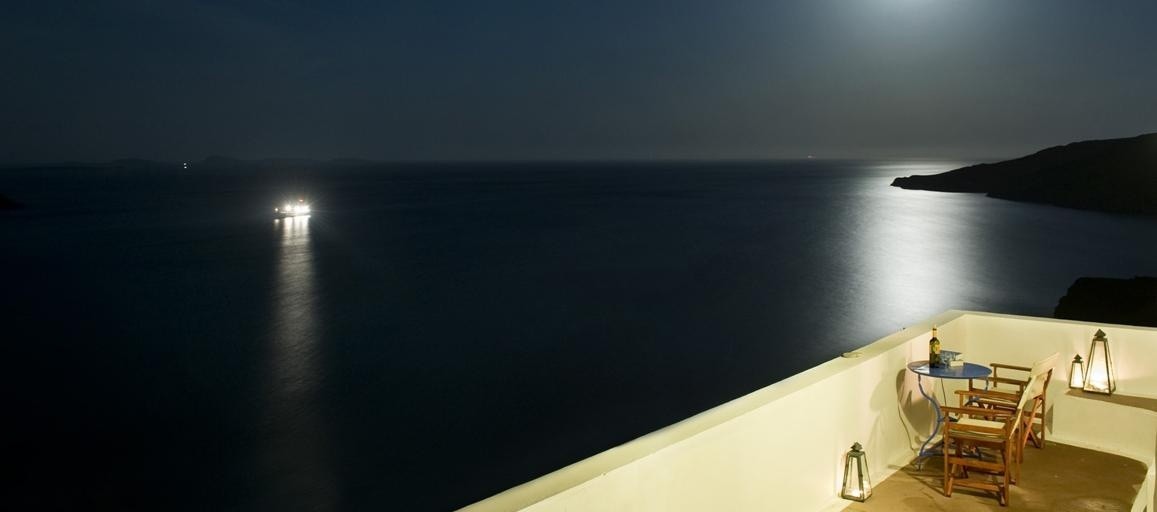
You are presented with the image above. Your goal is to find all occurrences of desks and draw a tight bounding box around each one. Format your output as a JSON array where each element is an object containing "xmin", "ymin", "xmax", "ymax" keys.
[{"xmin": 907, "ymin": 360, "xmax": 991, "ymax": 471}]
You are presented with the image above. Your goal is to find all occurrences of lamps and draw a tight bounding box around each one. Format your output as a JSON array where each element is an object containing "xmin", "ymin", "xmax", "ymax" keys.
[
  {"xmin": 839, "ymin": 441, "xmax": 872, "ymax": 502},
  {"xmin": 1068, "ymin": 328, "xmax": 1116, "ymax": 395}
]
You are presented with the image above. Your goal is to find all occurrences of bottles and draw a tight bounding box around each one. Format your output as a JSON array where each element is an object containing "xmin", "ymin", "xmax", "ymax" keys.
[{"xmin": 929, "ymin": 323, "xmax": 941, "ymax": 369}]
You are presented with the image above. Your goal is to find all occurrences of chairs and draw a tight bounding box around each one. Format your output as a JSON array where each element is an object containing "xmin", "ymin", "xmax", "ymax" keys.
[{"xmin": 938, "ymin": 352, "xmax": 1059, "ymax": 505}]
